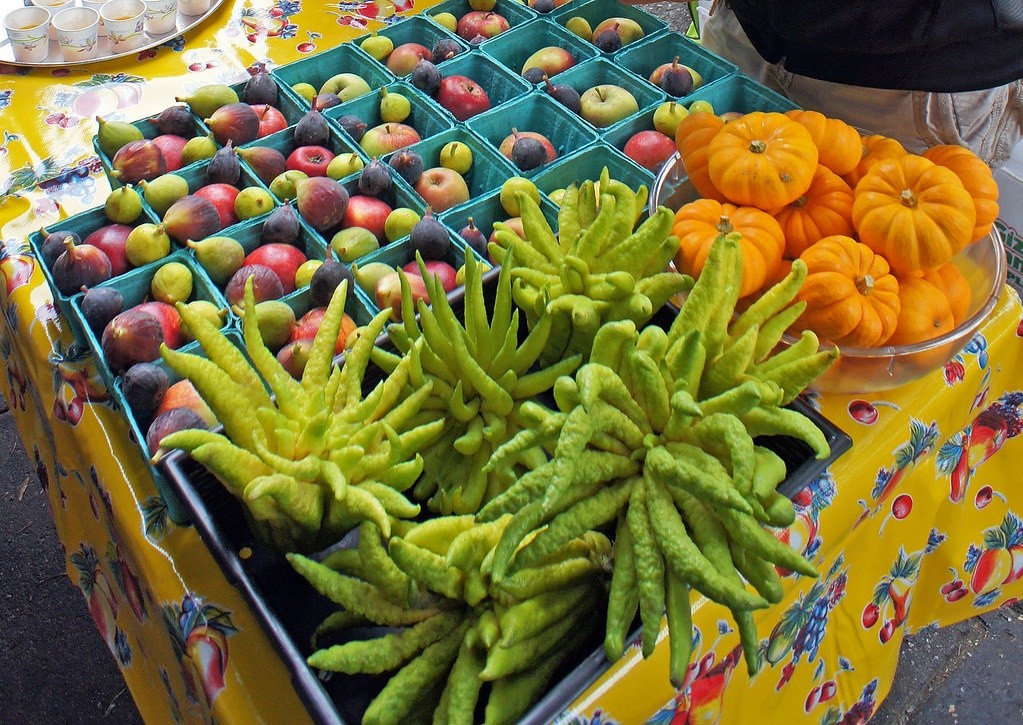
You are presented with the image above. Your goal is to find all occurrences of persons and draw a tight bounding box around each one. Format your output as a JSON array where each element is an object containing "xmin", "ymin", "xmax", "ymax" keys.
[{"xmin": 700, "ymin": 0, "xmax": 1023, "ymax": 178}]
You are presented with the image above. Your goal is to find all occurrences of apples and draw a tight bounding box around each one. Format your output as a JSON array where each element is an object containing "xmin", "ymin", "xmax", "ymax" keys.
[{"xmin": 85, "ymin": 0, "xmax": 750, "ymax": 429}]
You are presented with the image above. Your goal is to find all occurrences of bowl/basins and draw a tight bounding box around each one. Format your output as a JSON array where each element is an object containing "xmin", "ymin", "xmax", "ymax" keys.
[{"xmin": 645, "ymin": 149, "xmax": 1007, "ymax": 393}]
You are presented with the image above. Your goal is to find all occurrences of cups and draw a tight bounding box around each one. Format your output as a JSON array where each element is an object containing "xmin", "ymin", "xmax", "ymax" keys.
[
  {"xmin": 143, "ymin": 0, "xmax": 177, "ymax": 34},
  {"xmin": 177, "ymin": 0, "xmax": 210, "ymax": 16},
  {"xmin": 3, "ymin": 7, "xmax": 49, "ymax": 64},
  {"xmin": 30, "ymin": 0, "xmax": 75, "ymax": 41},
  {"xmin": 101, "ymin": 0, "xmax": 147, "ymax": 54},
  {"xmin": 82, "ymin": 0, "xmax": 108, "ymax": 36},
  {"xmin": 52, "ymin": 7, "xmax": 100, "ymax": 61}
]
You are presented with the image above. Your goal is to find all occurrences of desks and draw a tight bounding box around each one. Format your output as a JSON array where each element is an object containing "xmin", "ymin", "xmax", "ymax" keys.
[{"xmin": 0, "ymin": 0, "xmax": 1023, "ymax": 725}]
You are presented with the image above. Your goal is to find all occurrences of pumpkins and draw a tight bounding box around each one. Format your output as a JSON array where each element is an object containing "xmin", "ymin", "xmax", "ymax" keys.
[{"xmin": 670, "ymin": 110, "xmax": 999, "ymax": 376}]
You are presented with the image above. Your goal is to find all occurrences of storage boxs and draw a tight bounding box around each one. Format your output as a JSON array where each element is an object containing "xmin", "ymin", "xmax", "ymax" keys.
[{"xmin": 23, "ymin": 0, "xmax": 850, "ymax": 725}]
